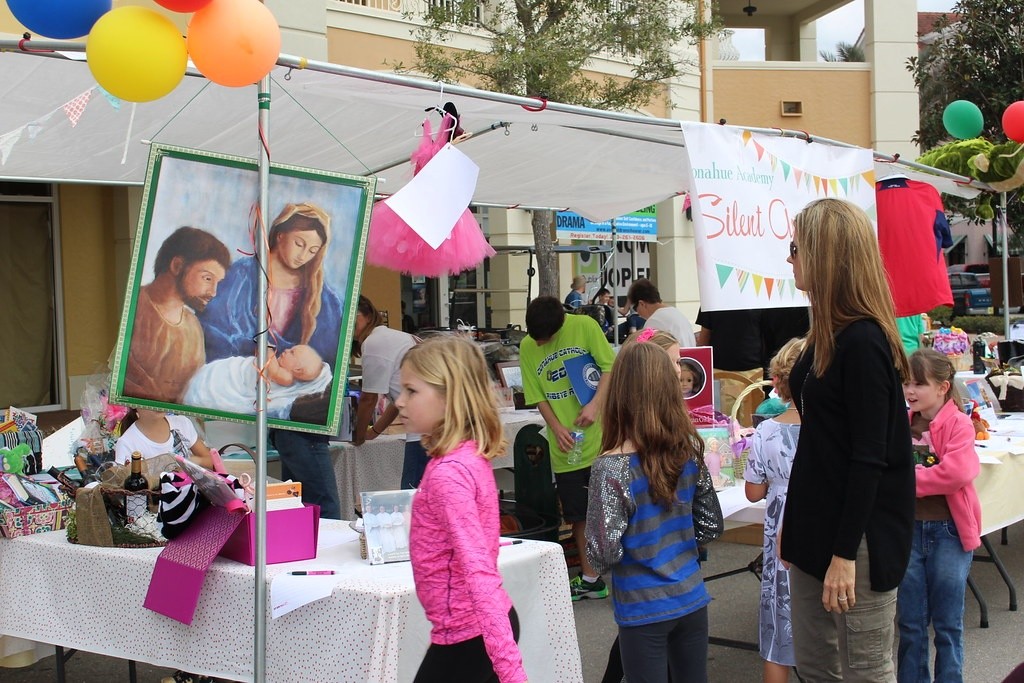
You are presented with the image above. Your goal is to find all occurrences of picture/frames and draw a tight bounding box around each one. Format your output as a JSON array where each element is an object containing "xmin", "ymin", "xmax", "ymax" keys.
[
  {"xmin": 495, "ymin": 360, "xmax": 522, "ymax": 388},
  {"xmin": 109, "ymin": 142, "xmax": 377, "ymax": 437}
]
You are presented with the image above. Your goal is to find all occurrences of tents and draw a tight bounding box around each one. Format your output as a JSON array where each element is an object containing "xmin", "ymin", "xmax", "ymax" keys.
[{"xmin": 0, "ymin": 34, "xmax": 1011, "ymax": 683}]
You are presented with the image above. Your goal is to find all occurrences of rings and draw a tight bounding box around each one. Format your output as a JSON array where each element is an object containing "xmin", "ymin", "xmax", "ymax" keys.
[{"xmin": 838, "ymin": 596, "xmax": 847, "ymax": 601}]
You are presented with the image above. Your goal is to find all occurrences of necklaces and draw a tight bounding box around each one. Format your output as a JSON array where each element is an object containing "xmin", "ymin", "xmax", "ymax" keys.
[{"xmin": 787, "ymin": 408, "xmax": 797, "ymax": 409}]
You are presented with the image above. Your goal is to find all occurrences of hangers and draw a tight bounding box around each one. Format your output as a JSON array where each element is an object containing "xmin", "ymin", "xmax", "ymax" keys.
[{"xmin": 413, "ymin": 80, "xmax": 457, "ymax": 137}]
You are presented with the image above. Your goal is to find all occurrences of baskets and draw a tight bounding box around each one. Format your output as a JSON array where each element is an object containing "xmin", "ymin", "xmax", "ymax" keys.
[
  {"xmin": 728, "ymin": 380, "xmax": 774, "ymax": 479},
  {"xmin": 979, "ymin": 340, "xmax": 1002, "ymax": 374},
  {"xmin": 945, "ymin": 354, "xmax": 972, "ymax": 371},
  {"xmin": 985, "ymin": 368, "xmax": 1024, "ymax": 412}
]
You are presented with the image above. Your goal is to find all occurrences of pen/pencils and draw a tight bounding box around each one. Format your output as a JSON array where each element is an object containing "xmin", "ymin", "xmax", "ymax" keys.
[
  {"xmin": 529, "ymin": 411, "xmax": 540, "ymax": 413},
  {"xmin": 975, "ymin": 444, "xmax": 988, "ymax": 448},
  {"xmin": 987, "ymin": 429, "xmax": 997, "ymax": 432},
  {"xmin": 287, "ymin": 571, "xmax": 335, "ymax": 575},
  {"xmin": 499, "ymin": 540, "xmax": 523, "ymax": 546}
]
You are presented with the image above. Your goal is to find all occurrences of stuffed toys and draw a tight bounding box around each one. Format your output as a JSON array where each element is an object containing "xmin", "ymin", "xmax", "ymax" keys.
[{"xmin": 904, "ymin": 136, "xmax": 1024, "ymax": 192}]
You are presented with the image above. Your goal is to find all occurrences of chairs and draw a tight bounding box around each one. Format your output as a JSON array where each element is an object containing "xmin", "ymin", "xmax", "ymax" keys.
[
  {"xmin": 985, "ymin": 372, "xmax": 1024, "ymax": 412},
  {"xmin": 498, "ymin": 424, "xmax": 561, "ymax": 542}
]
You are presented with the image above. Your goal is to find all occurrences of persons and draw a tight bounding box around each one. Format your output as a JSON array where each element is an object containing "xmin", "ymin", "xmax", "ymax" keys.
[
  {"xmin": 895, "ymin": 313, "xmax": 924, "ymax": 358},
  {"xmin": 350, "ymin": 294, "xmax": 432, "ymax": 490},
  {"xmin": 774, "ymin": 201, "xmax": 918, "ymax": 683},
  {"xmin": 896, "ymin": 348, "xmax": 982, "ymax": 683},
  {"xmin": 365, "ymin": 502, "xmax": 411, "ymax": 558},
  {"xmin": 114, "ymin": 408, "xmax": 215, "ymax": 466},
  {"xmin": 394, "ymin": 276, "xmax": 810, "ymax": 683},
  {"xmin": 268, "ymin": 428, "xmax": 341, "ymax": 520}
]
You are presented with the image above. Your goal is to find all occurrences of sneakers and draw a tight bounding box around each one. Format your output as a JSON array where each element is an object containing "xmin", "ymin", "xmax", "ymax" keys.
[{"xmin": 569, "ymin": 572, "xmax": 609, "ymax": 601}]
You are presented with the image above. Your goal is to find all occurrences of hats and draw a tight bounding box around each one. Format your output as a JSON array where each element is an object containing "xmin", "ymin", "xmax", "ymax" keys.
[{"xmin": 570, "ymin": 276, "xmax": 587, "ymax": 289}]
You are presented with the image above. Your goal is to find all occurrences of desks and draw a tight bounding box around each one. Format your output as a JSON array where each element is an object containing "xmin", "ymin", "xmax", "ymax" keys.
[
  {"xmin": 699, "ymin": 412, "xmax": 1024, "ymax": 651},
  {"xmin": 954, "ymin": 368, "xmax": 1001, "ymax": 409},
  {"xmin": 223, "ymin": 406, "xmax": 548, "ymax": 521}
]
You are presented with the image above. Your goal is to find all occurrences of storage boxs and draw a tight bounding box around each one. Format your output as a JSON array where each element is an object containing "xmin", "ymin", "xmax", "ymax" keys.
[
  {"xmin": 0, "ymin": 515, "xmax": 584, "ymax": 683},
  {"xmin": 143, "ymin": 502, "xmax": 321, "ymax": 626}
]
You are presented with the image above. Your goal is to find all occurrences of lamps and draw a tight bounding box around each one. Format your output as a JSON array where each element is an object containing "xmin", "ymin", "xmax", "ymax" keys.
[{"xmin": 743, "ymin": 0, "xmax": 757, "ymax": 16}]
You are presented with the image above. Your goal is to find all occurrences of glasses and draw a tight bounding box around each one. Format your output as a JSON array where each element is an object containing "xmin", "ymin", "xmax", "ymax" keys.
[
  {"xmin": 633, "ymin": 302, "xmax": 640, "ymax": 312},
  {"xmin": 789, "ymin": 240, "xmax": 799, "ymax": 259}
]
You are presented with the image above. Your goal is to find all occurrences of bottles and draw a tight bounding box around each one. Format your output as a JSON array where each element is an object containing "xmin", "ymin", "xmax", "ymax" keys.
[
  {"xmin": 45, "ymin": 456, "xmax": 125, "ymax": 529},
  {"xmin": 124, "ymin": 451, "xmax": 149, "ymax": 510},
  {"xmin": 567, "ymin": 425, "xmax": 585, "ymax": 466},
  {"xmin": 973, "ymin": 337, "xmax": 985, "ymax": 374}
]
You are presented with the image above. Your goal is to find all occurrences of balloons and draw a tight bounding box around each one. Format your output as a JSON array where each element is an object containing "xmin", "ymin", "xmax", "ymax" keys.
[
  {"xmin": 1002, "ymin": 101, "xmax": 1024, "ymax": 143},
  {"xmin": 942, "ymin": 100, "xmax": 984, "ymax": 140},
  {"xmin": 6, "ymin": 0, "xmax": 281, "ymax": 105}
]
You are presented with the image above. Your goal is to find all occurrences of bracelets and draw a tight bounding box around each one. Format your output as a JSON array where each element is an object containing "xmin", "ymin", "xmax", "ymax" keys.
[{"xmin": 372, "ymin": 426, "xmax": 381, "ymax": 435}]
